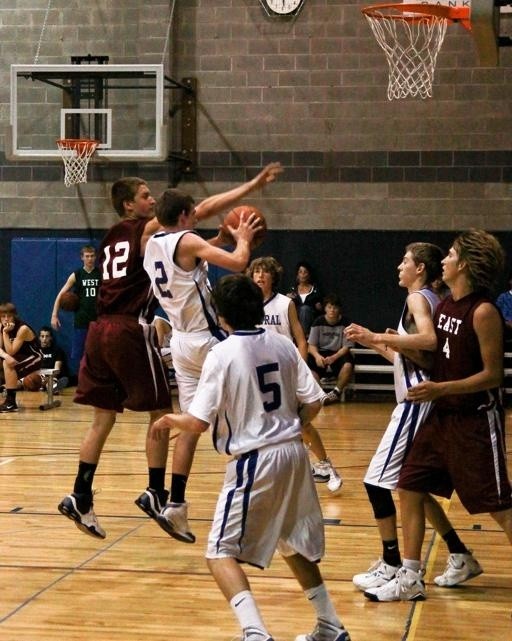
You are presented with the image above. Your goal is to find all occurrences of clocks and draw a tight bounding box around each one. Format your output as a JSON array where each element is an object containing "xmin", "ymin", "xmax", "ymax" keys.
[{"xmin": 257, "ymin": 0, "xmax": 306, "ymax": 19}]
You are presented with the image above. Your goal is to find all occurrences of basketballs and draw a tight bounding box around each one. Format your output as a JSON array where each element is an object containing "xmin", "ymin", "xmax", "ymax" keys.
[
  {"xmin": 23, "ymin": 372, "xmax": 42, "ymax": 392},
  {"xmin": 224, "ymin": 206, "xmax": 266, "ymax": 251},
  {"xmin": 60, "ymin": 292, "xmax": 78, "ymax": 312}
]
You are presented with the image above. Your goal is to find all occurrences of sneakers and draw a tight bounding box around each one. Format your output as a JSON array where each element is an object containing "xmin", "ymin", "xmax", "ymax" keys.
[
  {"xmin": 0, "ymin": 400, "xmax": 19, "ymax": 413},
  {"xmin": 326, "ymin": 385, "xmax": 342, "ymax": 403},
  {"xmin": 294, "ymin": 616, "xmax": 352, "ymax": 641},
  {"xmin": 46, "ymin": 376, "xmax": 68, "ymax": 395},
  {"xmin": 241, "ymin": 626, "xmax": 274, "ymax": 641},
  {"xmin": 432, "ymin": 547, "xmax": 485, "ymax": 590},
  {"xmin": 133, "ymin": 485, "xmax": 197, "ymax": 546},
  {"xmin": 57, "ymin": 492, "xmax": 109, "ymax": 541},
  {"xmin": 350, "ymin": 555, "xmax": 429, "ymax": 603},
  {"xmin": 309, "ymin": 459, "xmax": 342, "ymax": 494}
]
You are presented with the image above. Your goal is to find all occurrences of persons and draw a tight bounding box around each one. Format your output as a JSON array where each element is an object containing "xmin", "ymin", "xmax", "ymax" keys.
[
  {"xmin": 149, "ymin": 266, "xmax": 356, "ymax": 407},
  {"xmin": 247, "ymin": 256, "xmax": 343, "ymax": 491},
  {"xmin": 151, "ymin": 273, "xmax": 351, "ymax": 641},
  {"xmin": 364, "ymin": 228, "xmax": 512, "ymax": 603},
  {"xmin": 430, "ymin": 272, "xmax": 511, "ymax": 353},
  {"xmin": 1, "ymin": 248, "xmax": 100, "ymax": 411},
  {"xmin": 59, "ymin": 161, "xmax": 284, "ymax": 538},
  {"xmin": 343, "ymin": 243, "xmax": 484, "ymax": 592},
  {"xmin": 142, "ymin": 188, "xmax": 263, "ymax": 542}
]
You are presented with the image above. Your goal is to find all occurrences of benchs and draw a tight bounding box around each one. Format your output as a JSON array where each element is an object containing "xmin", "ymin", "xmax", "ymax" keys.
[
  {"xmin": 300, "ymin": 328, "xmax": 512, "ymax": 402},
  {"xmin": 35, "ymin": 368, "xmax": 62, "ymax": 411}
]
